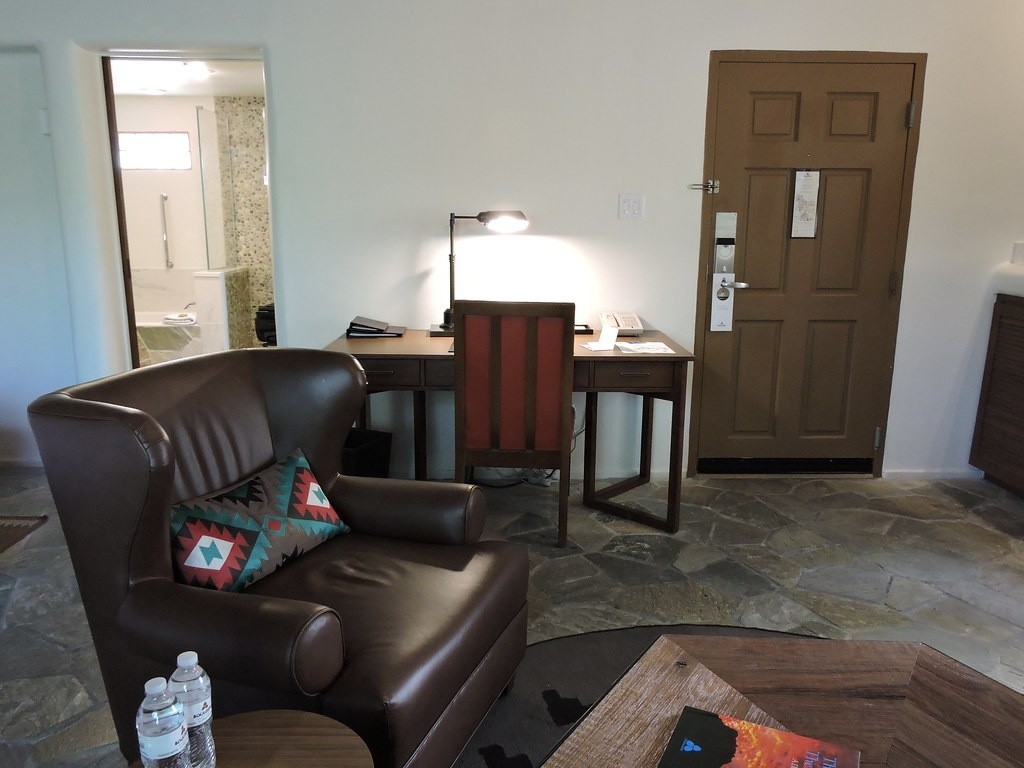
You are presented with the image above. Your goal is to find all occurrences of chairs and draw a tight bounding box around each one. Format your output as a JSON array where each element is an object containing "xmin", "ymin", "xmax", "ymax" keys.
[{"xmin": 445, "ymin": 299, "xmax": 574, "ymax": 546}]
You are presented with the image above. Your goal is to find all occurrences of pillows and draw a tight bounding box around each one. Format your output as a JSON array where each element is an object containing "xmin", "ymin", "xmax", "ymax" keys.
[{"xmin": 163, "ymin": 447, "xmax": 352, "ymax": 594}]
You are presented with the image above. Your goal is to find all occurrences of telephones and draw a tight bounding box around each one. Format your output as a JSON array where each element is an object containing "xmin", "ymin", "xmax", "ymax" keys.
[{"xmin": 600, "ymin": 311, "xmax": 644, "ymax": 337}]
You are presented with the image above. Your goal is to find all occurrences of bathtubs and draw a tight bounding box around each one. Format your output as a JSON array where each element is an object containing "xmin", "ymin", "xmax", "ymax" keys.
[{"xmin": 136, "ymin": 312, "xmax": 200, "ymax": 350}]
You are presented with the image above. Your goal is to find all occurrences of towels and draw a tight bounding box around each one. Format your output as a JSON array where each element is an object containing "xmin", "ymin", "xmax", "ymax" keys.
[{"xmin": 162, "ymin": 313, "xmax": 198, "ymax": 326}]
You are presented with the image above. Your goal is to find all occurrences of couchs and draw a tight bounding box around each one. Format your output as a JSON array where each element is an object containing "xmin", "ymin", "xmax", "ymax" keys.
[{"xmin": 27, "ymin": 342, "xmax": 532, "ymax": 768}]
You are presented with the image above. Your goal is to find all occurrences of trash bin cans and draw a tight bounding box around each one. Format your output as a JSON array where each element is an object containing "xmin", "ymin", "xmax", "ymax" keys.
[{"xmin": 343, "ymin": 426, "xmax": 392, "ymax": 478}]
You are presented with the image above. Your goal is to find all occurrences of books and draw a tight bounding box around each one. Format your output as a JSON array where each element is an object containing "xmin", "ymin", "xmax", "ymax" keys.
[{"xmin": 657, "ymin": 706, "xmax": 861, "ymax": 768}]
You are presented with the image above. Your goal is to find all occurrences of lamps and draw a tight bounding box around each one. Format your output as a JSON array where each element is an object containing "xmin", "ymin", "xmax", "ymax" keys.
[{"xmin": 427, "ymin": 210, "xmax": 529, "ymax": 338}]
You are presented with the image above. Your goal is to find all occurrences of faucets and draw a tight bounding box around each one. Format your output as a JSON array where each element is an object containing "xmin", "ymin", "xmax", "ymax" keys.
[{"xmin": 184, "ymin": 302, "xmax": 196, "ymax": 309}]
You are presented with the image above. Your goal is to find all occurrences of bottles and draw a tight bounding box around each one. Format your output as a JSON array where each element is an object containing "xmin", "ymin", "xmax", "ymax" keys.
[
  {"xmin": 166, "ymin": 650, "xmax": 217, "ymax": 768},
  {"xmin": 136, "ymin": 676, "xmax": 194, "ymax": 768}
]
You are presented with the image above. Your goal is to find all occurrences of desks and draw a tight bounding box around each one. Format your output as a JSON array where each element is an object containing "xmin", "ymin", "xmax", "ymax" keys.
[
  {"xmin": 124, "ymin": 710, "xmax": 376, "ymax": 768},
  {"xmin": 322, "ymin": 325, "xmax": 700, "ymax": 530},
  {"xmin": 536, "ymin": 628, "xmax": 1024, "ymax": 768}
]
